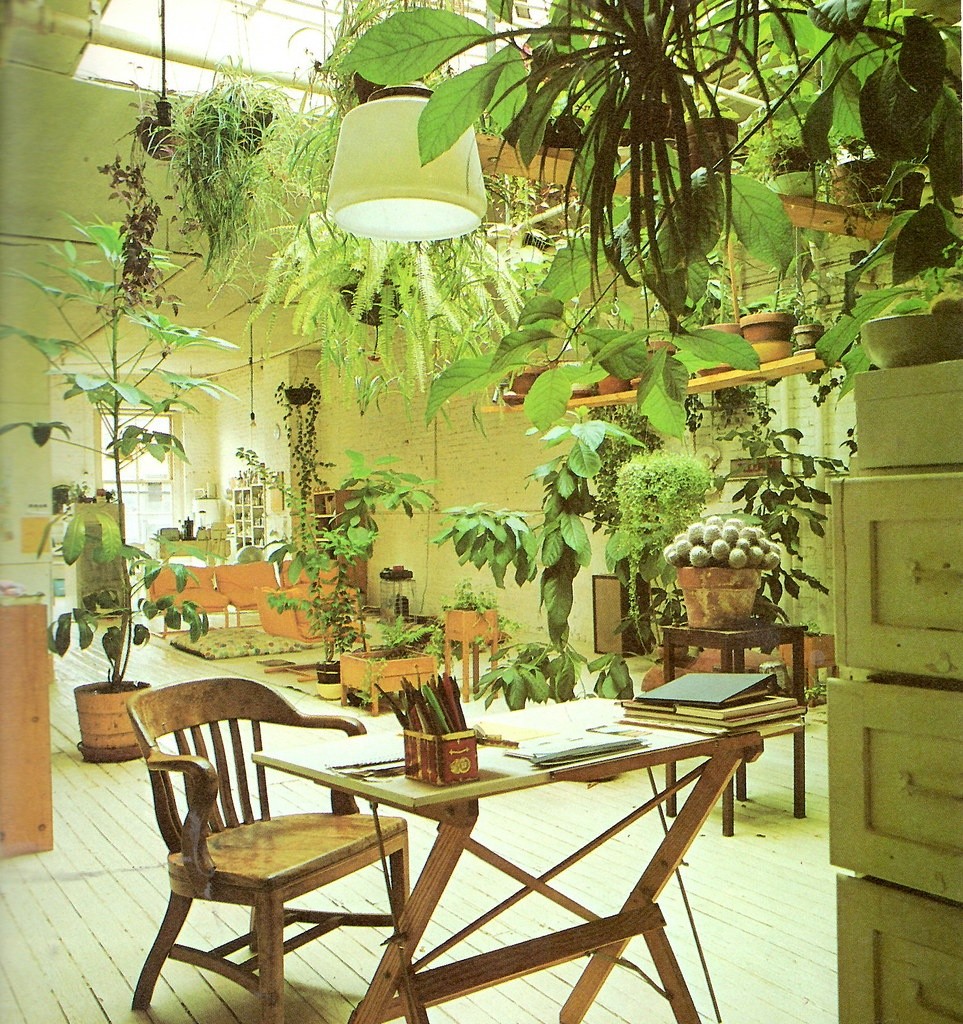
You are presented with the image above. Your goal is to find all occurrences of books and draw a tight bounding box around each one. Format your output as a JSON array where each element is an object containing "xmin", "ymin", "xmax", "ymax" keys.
[{"xmin": 615, "ymin": 694, "xmax": 807, "ymax": 735}]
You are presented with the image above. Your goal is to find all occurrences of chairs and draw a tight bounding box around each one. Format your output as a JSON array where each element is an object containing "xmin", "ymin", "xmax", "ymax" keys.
[
  {"xmin": 279, "ymin": 559, "xmax": 340, "ymax": 589},
  {"xmin": 214, "ymin": 561, "xmax": 279, "ymax": 627},
  {"xmin": 126, "ymin": 676, "xmax": 411, "ymax": 1024},
  {"xmin": 149, "ymin": 564, "xmax": 229, "ymax": 640}
]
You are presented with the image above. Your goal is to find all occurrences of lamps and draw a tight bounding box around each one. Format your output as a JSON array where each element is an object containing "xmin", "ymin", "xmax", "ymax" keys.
[
  {"xmin": 326, "ymin": 70, "xmax": 487, "ymax": 241},
  {"xmin": 249, "ymin": 324, "xmax": 257, "ymax": 430}
]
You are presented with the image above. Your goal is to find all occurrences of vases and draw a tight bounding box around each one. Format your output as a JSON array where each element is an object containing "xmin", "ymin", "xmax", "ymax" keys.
[
  {"xmin": 630, "ymin": 340, "xmax": 675, "ymax": 390},
  {"xmin": 557, "ymin": 361, "xmax": 594, "ymax": 399},
  {"xmin": 316, "ymin": 681, "xmax": 341, "ymax": 699},
  {"xmin": 597, "ymin": 376, "xmax": 629, "ymax": 395},
  {"xmin": 696, "ymin": 323, "xmax": 740, "ymax": 377},
  {"xmin": 502, "ymin": 366, "xmax": 549, "ymax": 406}
]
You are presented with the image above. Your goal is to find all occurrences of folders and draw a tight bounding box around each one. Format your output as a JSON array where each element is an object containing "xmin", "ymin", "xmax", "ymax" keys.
[{"xmin": 633, "ymin": 673, "xmax": 778, "ymax": 709}]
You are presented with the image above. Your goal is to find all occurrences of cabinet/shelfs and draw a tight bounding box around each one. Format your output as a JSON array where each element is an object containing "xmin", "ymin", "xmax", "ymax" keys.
[
  {"xmin": 0, "ymin": 602, "xmax": 54, "ymax": 860},
  {"xmin": 72, "ymin": 501, "xmax": 125, "ymax": 618},
  {"xmin": 192, "ymin": 498, "xmax": 221, "ymax": 537},
  {"xmin": 826, "ymin": 359, "xmax": 963, "ymax": 1024},
  {"xmin": 232, "ymin": 470, "xmax": 284, "ymax": 553},
  {"xmin": 313, "ymin": 489, "xmax": 367, "ymax": 615}
]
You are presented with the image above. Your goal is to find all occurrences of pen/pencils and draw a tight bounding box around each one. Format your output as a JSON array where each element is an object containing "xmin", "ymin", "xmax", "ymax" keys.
[{"xmin": 374, "ymin": 671, "xmax": 468, "ymax": 734}]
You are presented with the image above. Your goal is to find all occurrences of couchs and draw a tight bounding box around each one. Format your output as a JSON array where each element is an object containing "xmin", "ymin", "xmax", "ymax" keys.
[{"xmin": 253, "ymin": 586, "xmax": 366, "ymax": 644}]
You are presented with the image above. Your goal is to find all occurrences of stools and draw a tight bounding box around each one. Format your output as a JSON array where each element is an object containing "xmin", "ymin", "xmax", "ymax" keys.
[{"xmin": 659, "ymin": 624, "xmax": 809, "ymax": 837}]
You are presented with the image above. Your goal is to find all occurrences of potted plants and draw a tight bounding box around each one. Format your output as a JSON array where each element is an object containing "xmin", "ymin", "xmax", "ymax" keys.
[{"xmin": 0, "ymin": 0, "xmax": 963, "ymax": 767}]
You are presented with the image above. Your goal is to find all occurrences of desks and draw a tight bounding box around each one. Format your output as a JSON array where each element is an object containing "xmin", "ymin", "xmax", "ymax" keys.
[
  {"xmin": 251, "ymin": 718, "xmax": 809, "ymax": 1024},
  {"xmin": 159, "ymin": 538, "xmax": 232, "ymax": 565}
]
